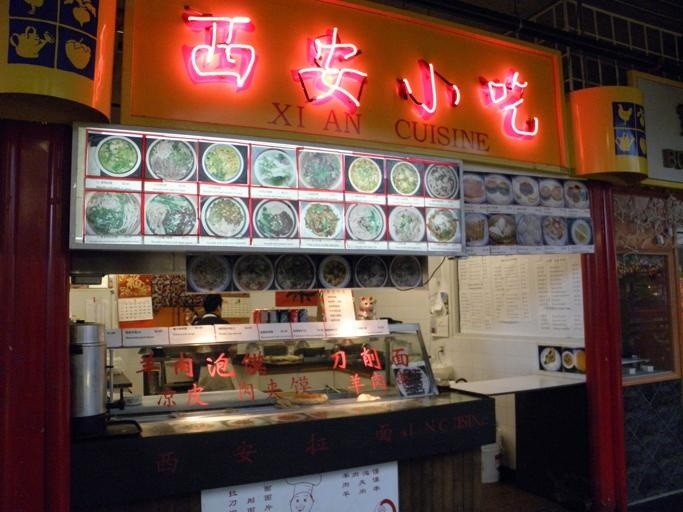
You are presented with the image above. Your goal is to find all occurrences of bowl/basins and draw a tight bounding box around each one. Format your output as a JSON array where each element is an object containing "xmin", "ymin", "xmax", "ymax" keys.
[{"xmin": 188, "ymin": 254, "xmax": 423, "ymax": 292}]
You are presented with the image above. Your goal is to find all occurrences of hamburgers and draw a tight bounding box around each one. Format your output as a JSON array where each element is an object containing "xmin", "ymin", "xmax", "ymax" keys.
[
  {"xmin": 498, "ymin": 182, "xmax": 510, "ymax": 195},
  {"xmin": 484, "ymin": 179, "xmax": 497, "ymax": 193}
]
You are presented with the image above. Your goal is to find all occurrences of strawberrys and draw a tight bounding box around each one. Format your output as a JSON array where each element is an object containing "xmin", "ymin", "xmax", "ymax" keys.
[{"xmin": 65, "ymin": 38, "xmax": 91, "ymax": 70}]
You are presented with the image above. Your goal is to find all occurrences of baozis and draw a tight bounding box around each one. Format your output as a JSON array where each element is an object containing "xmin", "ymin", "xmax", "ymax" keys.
[{"xmin": 517, "ymin": 214, "xmax": 541, "ymax": 244}]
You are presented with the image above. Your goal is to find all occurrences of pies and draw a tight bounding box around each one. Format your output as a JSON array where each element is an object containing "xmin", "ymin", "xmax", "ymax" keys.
[{"xmin": 289, "ymin": 392, "xmax": 329, "ymax": 405}]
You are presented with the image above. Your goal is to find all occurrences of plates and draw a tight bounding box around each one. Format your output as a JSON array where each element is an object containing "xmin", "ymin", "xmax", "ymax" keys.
[
  {"xmin": 87, "ymin": 191, "xmax": 142, "ymax": 236},
  {"xmin": 95, "ymin": 136, "xmax": 142, "ymax": 177},
  {"xmin": 424, "ymin": 164, "xmax": 459, "ymax": 199},
  {"xmin": 346, "ymin": 203, "xmax": 388, "ymax": 241},
  {"xmin": 301, "ymin": 201, "xmax": 344, "ymax": 239},
  {"xmin": 200, "ymin": 195, "xmax": 250, "ymax": 237},
  {"xmin": 389, "ymin": 161, "xmax": 422, "ymax": 195},
  {"xmin": 462, "ymin": 174, "xmax": 592, "ymax": 247},
  {"xmin": 144, "ymin": 193, "xmax": 198, "ymax": 235},
  {"xmin": 298, "ymin": 151, "xmax": 343, "ymax": 190},
  {"xmin": 539, "ymin": 347, "xmax": 586, "ymax": 373},
  {"xmin": 347, "ymin": 157, "xmax": 383, "ymax": 192},
  {"xmin": 144, "ymin": 138, "xmax": 197, "ymax": 181},
  {"xmin": 251, "ymin": 199, "xmax": 299, "ymax": 239},
  {"xmin": 426, "ymin": 209, "xmax": 458, "ymax": 243},
  {"xmin": 252, "ymin": 148, "xmax": 296, "ymax": 187},
  {"xmin": 200, "ymin": 142, "xmax": 244, "ymax": 184}
]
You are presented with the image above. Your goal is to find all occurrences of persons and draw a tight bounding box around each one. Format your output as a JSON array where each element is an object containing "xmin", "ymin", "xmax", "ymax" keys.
[
  {"xmin": 284, "ymin": 474, "xmax": 321, "ymax": 512},
  {"xmin": 197, "ymin": 293, "xmax": 232, "ymax": 369}
]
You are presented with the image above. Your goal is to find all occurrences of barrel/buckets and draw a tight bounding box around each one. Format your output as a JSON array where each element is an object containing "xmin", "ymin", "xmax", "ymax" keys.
[
  {"xmin": 69, "ymin": 323, "xmax": 108, "ymax": 420},
  {"xmin": 480, "ymin": 427, "xmax": 502, "ymax": 484}
]
